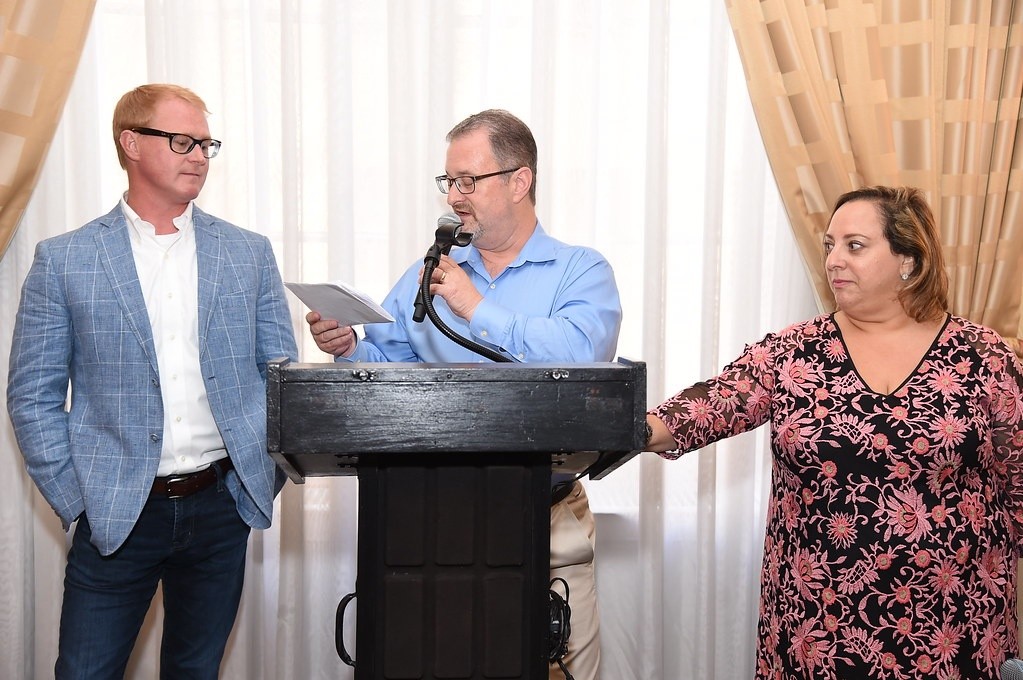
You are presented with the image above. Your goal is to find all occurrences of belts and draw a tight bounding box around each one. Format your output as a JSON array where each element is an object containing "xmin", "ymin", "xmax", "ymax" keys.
[{"xmin": 150, "ymin": 459, "xmax": 230, "ymax": 498}]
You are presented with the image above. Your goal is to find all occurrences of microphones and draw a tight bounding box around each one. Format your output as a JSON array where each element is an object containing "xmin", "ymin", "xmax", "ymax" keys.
[{"xmin": 413, "ymin": 213, "xmax": 462, "ymax": 323}]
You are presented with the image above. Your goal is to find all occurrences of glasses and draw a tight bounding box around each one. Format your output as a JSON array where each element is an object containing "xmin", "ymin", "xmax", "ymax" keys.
[
  {"xmin": 131, "ymin": 124, "xmax": 221, "ymax": 159},
  {"xmin": 435, "ymin": 167, "xmax": 520, "ymax": 195}
]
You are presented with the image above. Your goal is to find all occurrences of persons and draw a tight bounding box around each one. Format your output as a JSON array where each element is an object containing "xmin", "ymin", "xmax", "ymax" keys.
[
  {"xmin": 4, "ymin": 85, "xmax": 300, "ymax": 680},
  {"xmin": 303, "ymin": 109, "xmax": 620, "ymax": 680},
  {"xmin": 645, "ymin": 184, "xmax": 1023, "ymax": 680}
]
